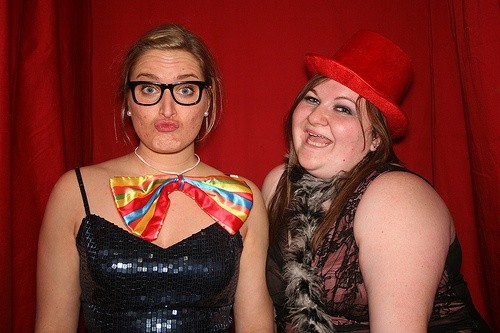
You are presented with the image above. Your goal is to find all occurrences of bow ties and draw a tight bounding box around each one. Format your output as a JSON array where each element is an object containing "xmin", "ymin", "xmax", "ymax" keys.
[{"xmin": 104, "ymin": 173, "xmax": 253, "ymax": 240}]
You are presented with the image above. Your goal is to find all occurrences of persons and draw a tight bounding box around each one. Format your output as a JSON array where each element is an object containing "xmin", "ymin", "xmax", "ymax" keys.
[
  {"xmin": 34, "ymin": 22, "xmax": 274, "ymax": 333},
  {"xmin": 258, "ymin": 29, "xmax": 493, "ymax": 332}
]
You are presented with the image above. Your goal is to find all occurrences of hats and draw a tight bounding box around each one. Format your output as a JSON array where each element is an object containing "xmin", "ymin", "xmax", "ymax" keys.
[{"xmin": 305, "ymin": 30, "xmax": 417, "ymax": 141}]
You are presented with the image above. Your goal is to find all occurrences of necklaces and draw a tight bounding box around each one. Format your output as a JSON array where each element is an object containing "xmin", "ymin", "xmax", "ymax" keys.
[{"xmin": 134, "ymin": 144, "xmax": 201, "ymax": 175}]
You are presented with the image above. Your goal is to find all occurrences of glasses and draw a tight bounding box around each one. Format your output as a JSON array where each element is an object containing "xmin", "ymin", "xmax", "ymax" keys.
[{"xmin": 127, "ymin": 80, "xmax": 211, "ymax": 105}]
